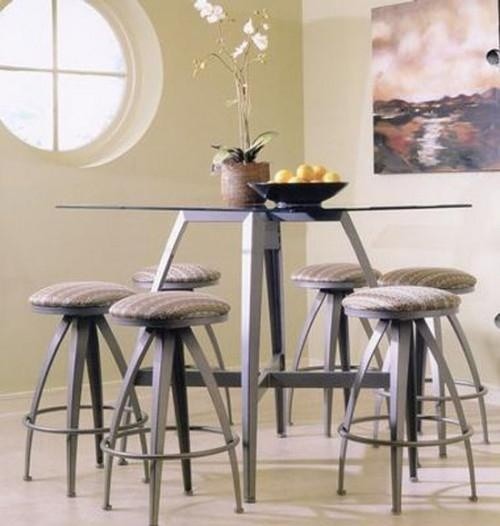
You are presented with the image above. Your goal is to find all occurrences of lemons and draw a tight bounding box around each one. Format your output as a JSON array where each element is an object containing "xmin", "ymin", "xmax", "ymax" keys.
[{"xmin": 266, "ymin": 164, "xmax": 340, "ymax": 183}]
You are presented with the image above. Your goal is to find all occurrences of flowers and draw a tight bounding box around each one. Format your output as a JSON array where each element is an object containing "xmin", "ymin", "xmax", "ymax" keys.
[{"xmin": 188, "ymin": 0, "xmax": 280, "ymax": 174}]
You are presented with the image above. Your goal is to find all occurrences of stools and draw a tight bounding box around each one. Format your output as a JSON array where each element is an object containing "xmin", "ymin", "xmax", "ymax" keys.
[
  {"xmin": 285, "ymin": 264, "xmax": 390, "ymax": 437},
  {"xmin": 372, "ymin": 266, "xmax": 490, "ymax": 466},
  {"xmin": 100, "ymin": 290, "xmax": 244, "ymax": 526},
  {"xmin": 336, "ymin": 284, "xmax": 477, "ymax": 515},
  {"xmin": 21, "ymin": 279, "xmax": 149, "ymax": 483},
  {"xmin": 117, "ymin": 263, "xmax": 233, "ymax": 466}
]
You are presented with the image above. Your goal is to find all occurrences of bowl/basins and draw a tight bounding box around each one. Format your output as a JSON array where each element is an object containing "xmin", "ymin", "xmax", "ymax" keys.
[{"xmin": 247, "ymin": 181, "xmax": 349, "ymax": 208}]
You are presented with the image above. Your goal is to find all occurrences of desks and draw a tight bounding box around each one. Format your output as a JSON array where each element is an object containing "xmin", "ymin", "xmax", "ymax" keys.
[{"xmin": 56, "ymin": 203, "xmax": 474, "ymax": 504}]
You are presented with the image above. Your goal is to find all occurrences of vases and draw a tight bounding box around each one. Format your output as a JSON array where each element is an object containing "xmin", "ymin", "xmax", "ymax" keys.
[{"xmin": 221, "ymin": 160, "xmax": 272, "ymax": 208}]
